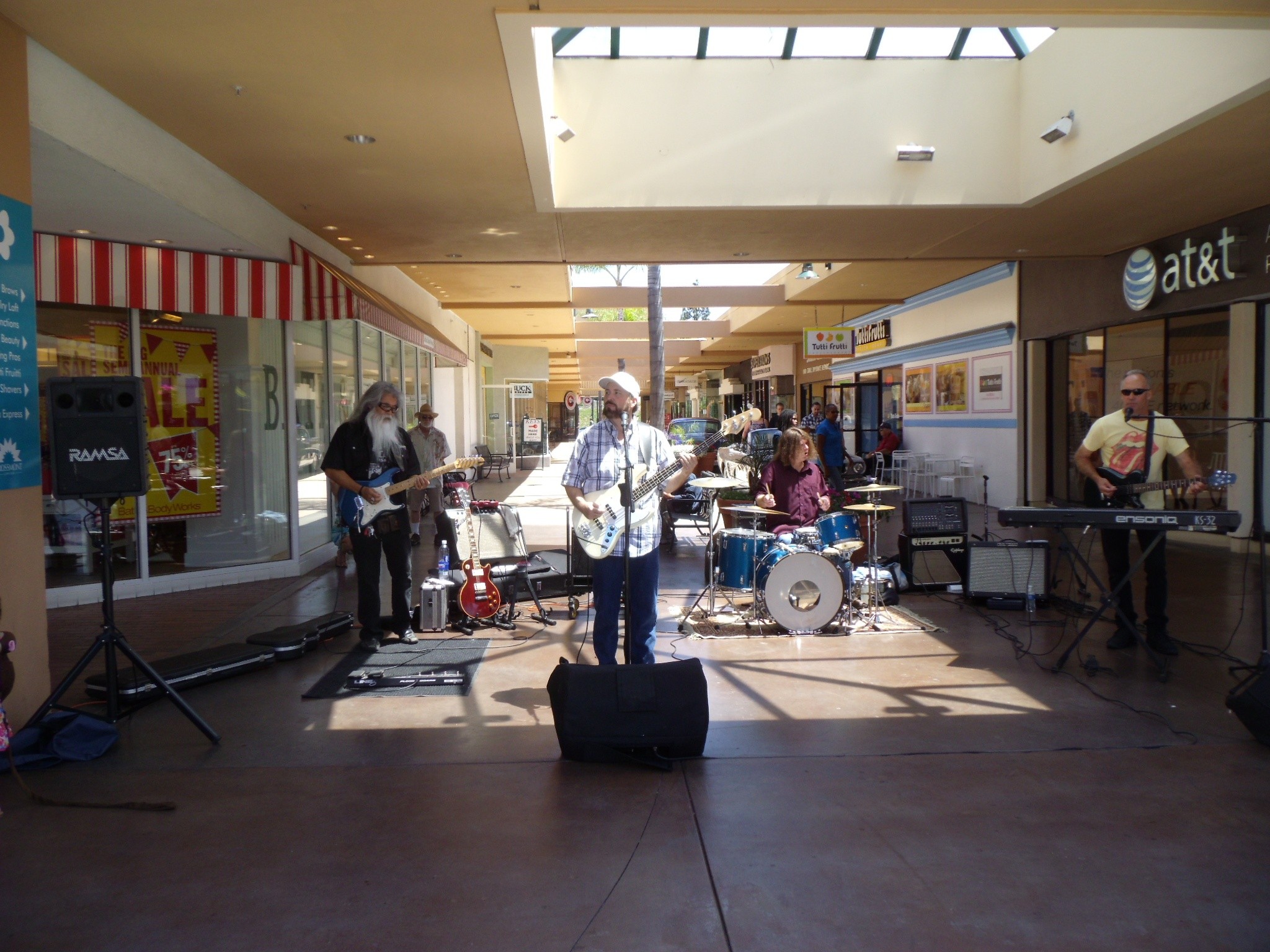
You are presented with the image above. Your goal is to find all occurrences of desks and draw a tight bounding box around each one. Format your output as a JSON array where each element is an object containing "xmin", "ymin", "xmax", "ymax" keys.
[
  {"xmin": 897, "ymin": 453, "xmax": 930, "ymax": 494},
  {"xmin": 924, "ymin": 455, "xmax": 962, "ymax": 501}
]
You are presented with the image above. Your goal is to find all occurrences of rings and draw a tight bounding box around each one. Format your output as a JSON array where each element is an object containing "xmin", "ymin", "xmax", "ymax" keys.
[{"xmin": 371, "ymin": 497, "xmax": 375, "ymax": 504}]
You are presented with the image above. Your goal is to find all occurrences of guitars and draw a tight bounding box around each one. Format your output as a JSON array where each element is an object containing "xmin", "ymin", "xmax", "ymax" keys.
[
  {"xmin": 565, "ymin": 400, "xmax": 764, "ymax": 561},
  {"xmin": 1083, "ymin": 466, "xmax": 1238, "ymax": 510},
  {"xmin": 455, "ymin": 486, "xmax": 501, "ymax": 620},
  {"xmin": 335, "ymin": 453, "xmax": 485, "ymax": 530}
]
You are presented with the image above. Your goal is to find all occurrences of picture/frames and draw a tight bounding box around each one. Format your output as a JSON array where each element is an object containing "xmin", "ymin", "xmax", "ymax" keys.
[
  {"xmin": 935, "ymin": 358, "xmax": 968, "ymax": 415},
  {"xmin": 905, "ymin": 363, "xmax": 933, "ymax": 415},
  {"xmin": 970, "ymin": 351, "xmax": 1014, "ymax": 415}
]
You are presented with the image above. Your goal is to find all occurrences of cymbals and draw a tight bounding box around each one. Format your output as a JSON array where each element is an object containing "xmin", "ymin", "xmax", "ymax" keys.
[
  {"xmin": 719, "ymin": 501, "xmax": 791, "ymax": 516},
  {"xmin": 843, "ymin": 502, "xmax": 896, "ymax": 513},
  {"xmin": 687, "ymin": 476, "xmax": 741, "ymax": 490},
  {"xmin": 844, "ymin": 482, "xmax": 904, "ymax": 493}
]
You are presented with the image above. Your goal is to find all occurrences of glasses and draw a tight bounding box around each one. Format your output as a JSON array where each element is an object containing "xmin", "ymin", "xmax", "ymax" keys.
[
  {"xmin": 378, "ymin": 402, "xmax": 399, "ymax": 414},
  {"xmin": 878, "ymin": 429, "xmax": 883, "ymax": 431},
  {"xmin": 421, "ymin": 415, "xmax": 433, "ymax": 419},
  {"xmin": 1120, "ymin": 389, "xmax": 1151, "ymax": 395},
  {"xmin": 829, "ymin": 411, "xmax": 839, "ymax": 414},
  {"xmin": 792, "ymin": 418, "xmax": 798, "ymax": 420}
]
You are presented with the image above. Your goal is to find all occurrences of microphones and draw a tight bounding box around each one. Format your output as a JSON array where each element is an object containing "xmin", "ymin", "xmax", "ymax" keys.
[
  {"xmin": 620, "ymin": 410, "xmax": 629, "ymax": 433},
  {"xmin": 1122, "ymin": 406, "xmax": 1134, "ymax": 422}
]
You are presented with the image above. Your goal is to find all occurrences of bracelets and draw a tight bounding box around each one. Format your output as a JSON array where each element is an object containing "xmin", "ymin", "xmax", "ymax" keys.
[{"xmin": 359, "ymin": 486, "xmax": 365, "ymax": 496}]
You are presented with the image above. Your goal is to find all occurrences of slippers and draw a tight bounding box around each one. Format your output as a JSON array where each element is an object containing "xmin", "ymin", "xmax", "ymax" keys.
[{"xmin": 335, "ymin": 556, "xmax": 347, "ymax": 568}]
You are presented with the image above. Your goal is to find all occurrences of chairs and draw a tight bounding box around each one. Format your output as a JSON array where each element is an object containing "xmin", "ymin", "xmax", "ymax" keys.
[
  {"xmin": 441, "ymin": 461, "xmax": 477, "ymax": 508},
  {"xmin": 475, "ymin": 444, "xmax": 512, "ymax": 481},
  {"xmin": 874, "ymin": 451, "xmax": 903, "ymax": 495},
  {"xmin": 938, "ymin": 456, "xmax": 981, "ymax": 505},
  {"xmin": 891, "ymin": 449, "xmax": 917, "ymax": 494},
  {"xmin": 904, "ymin": 455, "xmax": 937, "ymax": 501}
]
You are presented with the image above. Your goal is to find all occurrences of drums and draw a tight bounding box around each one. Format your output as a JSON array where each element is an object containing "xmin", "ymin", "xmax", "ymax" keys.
[
  {"xmin": 753, "ymin": 542, "xmax": 846, "ymax": 634},
  {"xmin": 813, "ymin": 511, "xmax": 866, "ymax": 554},
  {"xmin": 715, "ymin": 526, "xmax": 778, "ymax": 592},
  {"xmin": 792, "ymin": 526, "xmax": 823, "ymax": 547}
]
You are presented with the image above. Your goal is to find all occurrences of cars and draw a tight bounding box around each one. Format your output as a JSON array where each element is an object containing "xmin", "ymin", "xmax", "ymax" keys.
[
  {"xmin": 718, "ymin": 429, "xmax": 866, "ymax": 492},
  {"xmin": 667, "ymin": 417, "xmax": 721, "ymax": 452}
]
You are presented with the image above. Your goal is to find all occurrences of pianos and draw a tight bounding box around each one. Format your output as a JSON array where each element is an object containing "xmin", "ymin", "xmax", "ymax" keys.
[{"xmin": 997, "ymin": 504, "xmax": 1243, "ymax": 535}]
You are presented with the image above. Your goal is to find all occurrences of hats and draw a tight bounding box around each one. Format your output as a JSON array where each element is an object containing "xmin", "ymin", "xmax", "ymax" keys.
[
  {"xmin": 878, "ymin": 422, "xmax": 892, "ymax": 428},
  {"xmin": 414, "ymin": 403, "xmax": 439, "ymax": 418},
  {"xmin": 598, "ymin": 371, "xmax": 641, "ymax": 400}
]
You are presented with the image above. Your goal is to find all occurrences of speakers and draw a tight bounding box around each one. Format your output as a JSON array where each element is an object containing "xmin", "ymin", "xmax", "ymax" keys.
[
  {"xmin": 1226, "ymin": 655, "xmax": 1270, "ymax": 748},
  {"xmin": 679, "ymin": 488, "xmax": 752, "ymax": 632},
  {"xmin": 48, "ymin": 373, "xmax": 151, "ymax": 500},
  {"xmin": 902, "ymin": 495, "xmax": 969, "ymax": 537},
  {"xmin": 968, "ymin": 540, "xmax": 1052, "ymax": 602},
  {"xmin": 547, "ymin": 658, "xmax": 709, "ymax": 760},
  {"xmin": 715, "ymin": 514, "xmax": 781, "ymax": 638}
]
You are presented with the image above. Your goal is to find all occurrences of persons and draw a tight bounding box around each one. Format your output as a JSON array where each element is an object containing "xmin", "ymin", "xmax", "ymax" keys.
[
  {"xmin": 561, "ymin": 371, "xmax": 698, "ymax": 664},
  {"xmin": 1071, "ymin": 398, "xmax": 1092, "ymax": 433},
  {"xmin": 757, "ymin": 428, "xmax": 829, "ymax": 544},
  {"xmin": 1075, "ymin": 369, "xmax": 1202, "ymax": 654},
  {"xmin": 320, "ymin": 380, "xmax": 430, "ymax": 650},
  {"xmin": 865, "ymin": 423, "xmax": 900, "ymax": 468},
  {"xmin": 741, "ymin": 401, "xmax": 855, "ymax": 496},
  {"xmin": 407, "ymin": 404, "xmax": 451, "ymax": 548}
]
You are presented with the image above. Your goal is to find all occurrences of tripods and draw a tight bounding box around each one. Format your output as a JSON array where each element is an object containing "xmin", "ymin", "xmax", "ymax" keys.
[
  {"xmin": 12, "ymin": 501, "xmax": 224, "ymax": 746},
  {"xmin": 847, "ymin": 492, "xmax": 927, "ymax": 636}
]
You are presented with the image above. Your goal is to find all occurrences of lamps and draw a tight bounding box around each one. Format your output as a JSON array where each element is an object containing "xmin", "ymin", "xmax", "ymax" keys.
[
  {"xmin": 897, "ymin": 142, "xmax": 935, "ymax": 164},
  {"xmin": 795, "ymin": 263, "xmax": 821, "ymax": 280},
  {"xmin": 1043, "ymin": 115, "xmax": 1072, "ymax": 146},
  {"xmin": 581, "ymin": 309, "xmax": 599, "ymax": 319},
  {"xmin": 549, "ymin": 115, "xmax": 577, "ymax": 145}
]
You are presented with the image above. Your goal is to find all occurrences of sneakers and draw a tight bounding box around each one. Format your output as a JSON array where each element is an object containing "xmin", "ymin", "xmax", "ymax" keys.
[
  {"xmin": 434, "ymin": 534, "xmax": 440, "ymax": 545},
  {"xmin": 1147, "ymin": 632, "xmax": 1178, "ymax": 654},
  {"xmin": 361, "ymin": 638, "xmax": 381, "ymax": 653},
  {"xmin": 409, "ymin": 532, "xmax": 420, "ymax": 545},
  {"xmin": 399, "ymin": 627, "xmax": 419, "ymax": 644},
  {"xmin": 1106, "ymin": 628, "xmax": 1137, "ymax": 649}
]
